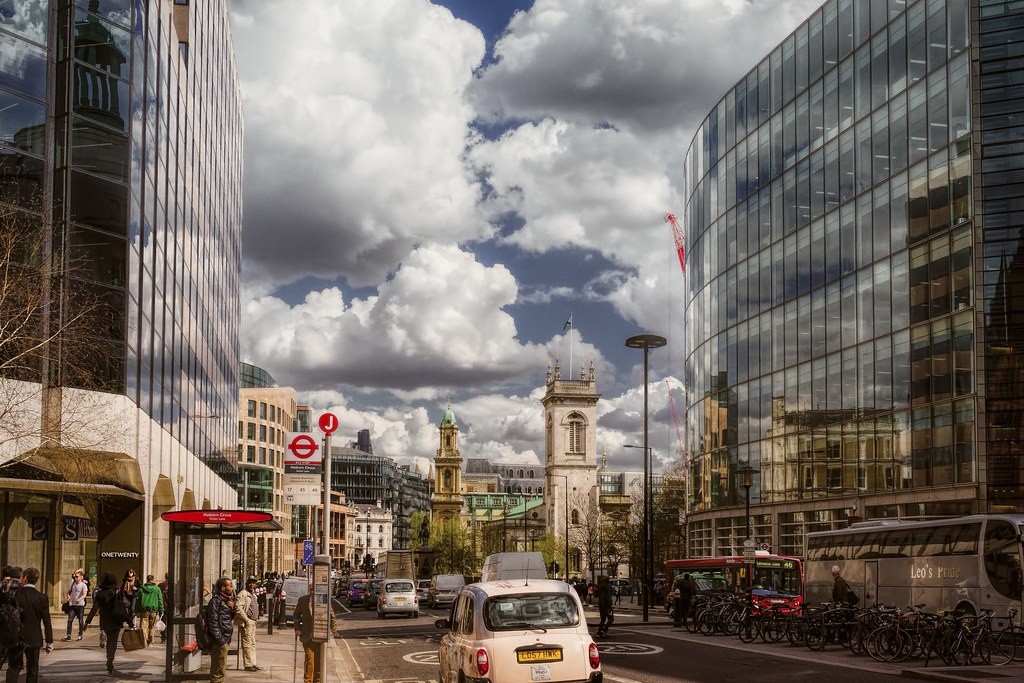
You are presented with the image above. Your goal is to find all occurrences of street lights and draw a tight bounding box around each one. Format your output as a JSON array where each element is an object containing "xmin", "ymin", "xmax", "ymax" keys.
[
  {"xmin": 545, "ymin": 473, "xmax": 568, "ymax": 583},
  {"xmin": 623, "ymin": 445, "xmax": 654, "ymax": 610},
  {"xmin": 624, "ymin": 334, "xmax": 667, "ymax": 621}
]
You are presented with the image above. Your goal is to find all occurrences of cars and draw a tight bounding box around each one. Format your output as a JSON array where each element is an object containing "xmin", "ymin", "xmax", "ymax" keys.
[
  {"xmin": 434, "ymin": 578, "xmax": 604, "ymax": 683},
  {"xmin": 376, "ymin": 578, "xmax": 424, "ymax": 619},
  {"xmin": 413, "ymin": 578, "xmax": 432, "ymax": 604},
  {"xmin": 278, "ymin": 575, "xmax": 308, "ymax": 621},
  {"xmin": 331, "ymin": 568, "xmax": 384, "ymax": 611}
]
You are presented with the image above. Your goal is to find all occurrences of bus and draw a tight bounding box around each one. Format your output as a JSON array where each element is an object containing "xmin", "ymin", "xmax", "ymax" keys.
[
  {"xmin": 803, "ymin": 513, "xmax": 1024, "ymax": 640},
  {"xmin": 664, "ymin": 549, "xmax": 804, "ymax": 623}
]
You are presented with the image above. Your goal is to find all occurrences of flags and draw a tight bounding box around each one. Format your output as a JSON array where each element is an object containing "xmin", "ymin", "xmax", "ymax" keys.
[{"xmin": 562, "ymin": 316, "xmax": 570, "ymax": 331}]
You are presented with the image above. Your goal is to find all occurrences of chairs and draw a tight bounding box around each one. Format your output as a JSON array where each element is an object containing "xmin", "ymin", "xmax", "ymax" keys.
[{"xmin": 522, "ymin": 602, "xmax": 561, "ymax": 622}]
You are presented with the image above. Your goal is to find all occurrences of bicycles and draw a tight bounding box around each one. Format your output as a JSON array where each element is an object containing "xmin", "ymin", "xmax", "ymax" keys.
[{"xmin": 684, "ymin": 590, "xmax": 1024, "ymax": 668}]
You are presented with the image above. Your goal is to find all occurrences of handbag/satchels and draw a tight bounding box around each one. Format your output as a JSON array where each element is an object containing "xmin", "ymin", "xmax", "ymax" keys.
[
  {"xmin": 847, "ymin": 591, "xmax": 859, "ymax": 605},
  {"xmin": 122, "ymin": 627, "xmax": 145, "ymax": 651},
  {"xmin": 62, "ymin": 602, "xmax": 69, "ymax": 611},
  {"xmin": 155, "ymin": 618, "xmax": 166, "ymax": 631},
  {"xmin": 133, "ymin": 577, "xmax": 138, "ymax": 597},
  {"xmin": 113, "ymin": 591, "xmax": 127, "ymax": 620}
]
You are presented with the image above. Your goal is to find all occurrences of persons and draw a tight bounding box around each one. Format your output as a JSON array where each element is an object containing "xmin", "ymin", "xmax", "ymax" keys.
[
  {"xmin": 574, "ymin": 573, "xmax": 693, "ymax": 638},
  {"xmin": 0, "ymin": 565, "xmax": 169, "ymax": 683},
  {"xmin": 206, "ymin": 578, "xmax": 236, "ymax": 683},
  {"xmin": 418, "ymin": 517, "xmax": 429, "ymax": 539},
  {"xmin": 234, "ymin": 576, "xmax": 262, "ymax": 671},
  {"xmin": 830, "ymin": 566, "xmax": 848, "ymax": 603},
  {"xmin": 294, "ymin": 584, "xmax": 337, "ymax": 683}
]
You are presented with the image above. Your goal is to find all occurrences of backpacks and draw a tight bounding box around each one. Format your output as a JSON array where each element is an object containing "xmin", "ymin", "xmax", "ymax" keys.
[
  {"xmin": 0, "ymin": 589, "xmax": 23, "ymax": 649},
  {"xmin": 194, "ymin": 597, "xmax": 222, "ymax": 650}
]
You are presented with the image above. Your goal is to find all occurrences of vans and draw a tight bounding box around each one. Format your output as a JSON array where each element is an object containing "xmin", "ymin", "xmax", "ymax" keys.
[
  {"xmin": 609, "ymin": 579, "xmax": 633, "ymax": 596},
  {"xmin": 478, "ymin": 551, "xmax": 549, "ymax": 582},
  {"xmin": 427, "ymin": 572, "xmax": 467, "ymax": 609}
]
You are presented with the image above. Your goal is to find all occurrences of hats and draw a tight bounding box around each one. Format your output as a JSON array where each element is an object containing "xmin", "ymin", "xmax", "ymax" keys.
[{"xmin": 248, "ymin": 575, "xmax": 257, "ymax": 582}]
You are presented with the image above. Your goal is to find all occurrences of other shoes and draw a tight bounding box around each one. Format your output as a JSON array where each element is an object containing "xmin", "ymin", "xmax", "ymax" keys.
[
  {"xmin": 161, "ymin": 640, "xmax": 165, "ymax": 644},
  {"xmin": 597, "ymin": 632, "xmax": 607, "ymax": 639},
  {"xmin": 252, "ymin": 665, "xmax": 264, "ymax": 670},
  {"xmin": 245, "ymin": 667, "xmax": 257, "ymax": 671},
  {"xmin": 100, "ymin": 636, "xmax": 106, "ymax": 648},
  {"xmin": 109, "ymin": 670, "xmax": 124, "ymax": 677},
  {"xmin": 78, "ymin": 635, "xmax": 82, "ymax": 641},
  {"xmin": 601, "ymin": 630, "xmax": 609, "ymax": 637},
  {"xmin": 148, "ymin": 644, "xmax": 154, "ymax": 647},
  {"xmin": 61, "ymin": 636, "xmax": 71, "ymax": 642}
]
